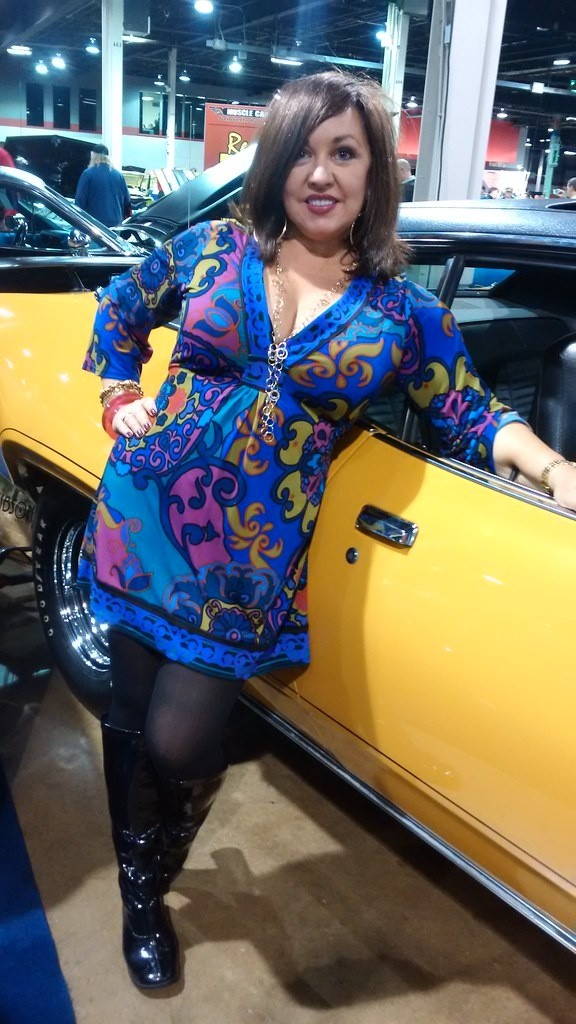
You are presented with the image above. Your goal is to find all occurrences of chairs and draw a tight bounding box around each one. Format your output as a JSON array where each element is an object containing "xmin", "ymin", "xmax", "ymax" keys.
[{"xmin": 529, "ymin": 332, "xmax": 576, "ymax": 465}]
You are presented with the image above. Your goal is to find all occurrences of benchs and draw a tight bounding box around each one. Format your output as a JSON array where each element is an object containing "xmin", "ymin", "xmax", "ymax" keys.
[{"xmin": 363, "ymin": 308, "xmax": 568, "ymax": 467}]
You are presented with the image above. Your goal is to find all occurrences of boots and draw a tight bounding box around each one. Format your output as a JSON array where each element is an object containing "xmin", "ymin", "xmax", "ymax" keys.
[
  {"xmin": 161, "ymin": 763, "xmax": 232, "ymax": 894},
  {"xmin": 100, "ymin": 720, "xmax": 183, "ymax": 988}
]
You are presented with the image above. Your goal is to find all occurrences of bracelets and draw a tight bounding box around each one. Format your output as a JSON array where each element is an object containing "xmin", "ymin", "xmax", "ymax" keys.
[
  {"xmin": 540, "ymin": 456, "xmax": 575, "ymax": 495},
  {"xmin": 99, "ymin": 379, "xmax": 144, "ymax": 407},
  {"xmin": 106, "ymin": 396, "xmax": 140, "ymax": 440},
  {"xmin": 102, "ymin": 392, "xmax": 140, "ymax": 431}
]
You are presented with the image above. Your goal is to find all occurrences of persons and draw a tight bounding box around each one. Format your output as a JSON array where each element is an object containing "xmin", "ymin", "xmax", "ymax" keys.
[
  {"xmin": 0, "ymin": 147, "xmax": 19, "ymax": 213},
  {"xmin": 74, "ymin": 71, "xmax": 575, "ymax": 999}
]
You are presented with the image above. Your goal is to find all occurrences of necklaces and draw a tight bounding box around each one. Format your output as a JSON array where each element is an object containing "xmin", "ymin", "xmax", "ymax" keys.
[{"xmin": 259, "ymin": 240, "xmax": 359, "ymax": 440}]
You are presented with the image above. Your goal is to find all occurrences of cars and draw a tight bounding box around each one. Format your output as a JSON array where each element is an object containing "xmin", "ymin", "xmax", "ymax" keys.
[{"xmin": 0, "ymin": 131, "xmax": 576, "ymax": 956}]
